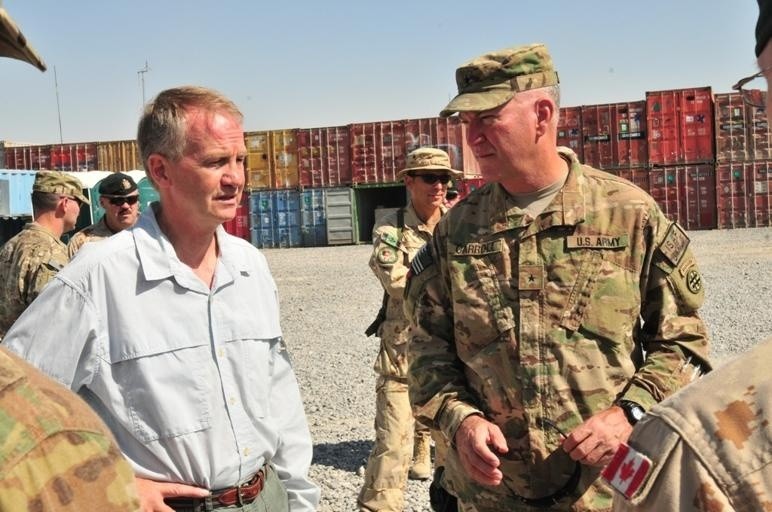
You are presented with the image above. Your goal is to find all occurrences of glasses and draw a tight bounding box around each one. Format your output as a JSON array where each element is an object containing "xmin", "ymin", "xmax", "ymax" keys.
[
  {"xmin": 446, "ymin": 192, "xmax": 458, "ymax": 201},
  {"xmin": 101, "ymin": 197, "xmax": 140, "ymax": 207},
  {"xmin": 493, "ymin": 421, "xmax": 583, "ymax": 511},
  {"xmin": 409, "ymin": 173, "xmax": 451, "ymax": 184},
  {"xmin": 733, "ymin": 68, "xmax": 771, "ymax": 113}
]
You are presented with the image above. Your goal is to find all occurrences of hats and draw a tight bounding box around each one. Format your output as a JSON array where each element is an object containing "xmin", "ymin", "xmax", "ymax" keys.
[
  {"xmin": 31, "ymin": 171, "xmax": 92, "ymax": 207},
  {"xmin": 437, "ymin": 43, "xmax": 563, "ymax": 119},
  {"xmin": 98, "ymin": 173, "xmax": 138, "ymax": 196},
  {"xmin": 397, "ymin": 146, "xmax": 464, "ymax": 191}
]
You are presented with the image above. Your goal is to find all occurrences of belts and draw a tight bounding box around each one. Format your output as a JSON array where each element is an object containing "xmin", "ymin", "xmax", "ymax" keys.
[{"xmin": 172, "ymin": 458, "xmax": 272, "ymax": 512}]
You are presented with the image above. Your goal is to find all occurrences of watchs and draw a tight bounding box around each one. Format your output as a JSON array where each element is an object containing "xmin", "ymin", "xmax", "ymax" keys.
[{"xmin": 617, "ymin": 397, "xmax": 643, "ymax": 429}]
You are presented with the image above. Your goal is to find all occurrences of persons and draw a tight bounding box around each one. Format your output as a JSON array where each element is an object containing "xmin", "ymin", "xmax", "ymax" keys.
[
  {"xmin": 404, "ymin": 42, "xmax": 710, "ymax": 512},
  {"xmin": 0, "ymin": 9, "xmax": 143, "ymax": 512},
  {"xmin": 0, "ymin": 170, "xmax": 92, "ymax": 340},
  {"xmin": 566, "ymin": 0, "xmax": 772, "ymax": 512},
  {"xmin": 68, "ymin": 173, "xmax": 138, "ymax": 255},
  {"xmin": 0, "ymin": 86, "xmax": 320, "ymax": 512},
  {"xmin": 357, "ymin": 149, "xmax": 468, "ymax": 512}
]
